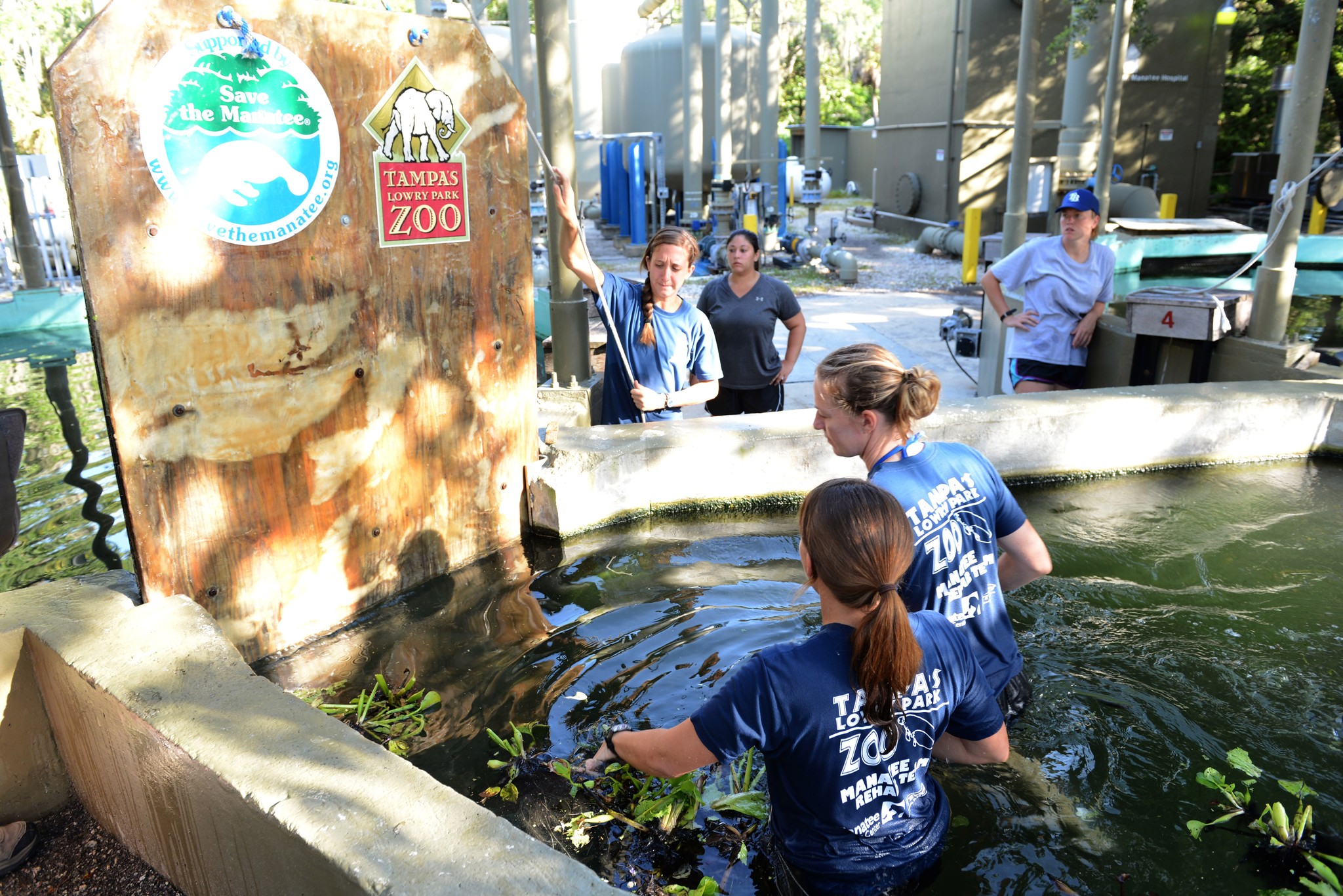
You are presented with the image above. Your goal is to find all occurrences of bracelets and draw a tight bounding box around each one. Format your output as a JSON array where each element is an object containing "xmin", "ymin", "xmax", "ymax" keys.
[{"xmin": 1001, "ymin": 309, "xmax": 1017, "ymax": 321}]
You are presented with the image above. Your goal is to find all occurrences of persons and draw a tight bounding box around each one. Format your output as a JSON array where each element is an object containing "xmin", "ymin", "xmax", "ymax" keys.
[
  {"xmin": 549, "ymin": 166, "xmax": 724, "ymax": 424},
  {"xmin": 576, "ymin": 478, "xmax": 1009, "ymax": 895},
  {"xmin": 979, "ymin": 189, "xmax": 1116, "ymax": 394},
  {"xmin": 696, "ymin": 229, "xmax": 806, "ymax": 416},
  {"xmin": 812, "ymin": 344, "xmax": 1053, "ymax": 723}
]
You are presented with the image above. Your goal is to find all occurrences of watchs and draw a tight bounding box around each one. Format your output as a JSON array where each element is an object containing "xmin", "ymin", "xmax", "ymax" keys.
[
  {"xmin": 664, "ymin": 393, "xmax": 674, "ymax": 409},
  {"xmin": 604, "ymin": 722, "xmax": 636, "ymax": 758}
]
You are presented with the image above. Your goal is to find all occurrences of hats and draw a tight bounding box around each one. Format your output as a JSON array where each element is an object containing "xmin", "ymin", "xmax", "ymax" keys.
[{"xmin": 1054, "ymin": 188, "xmax": 1100, "ymax": 216}]
